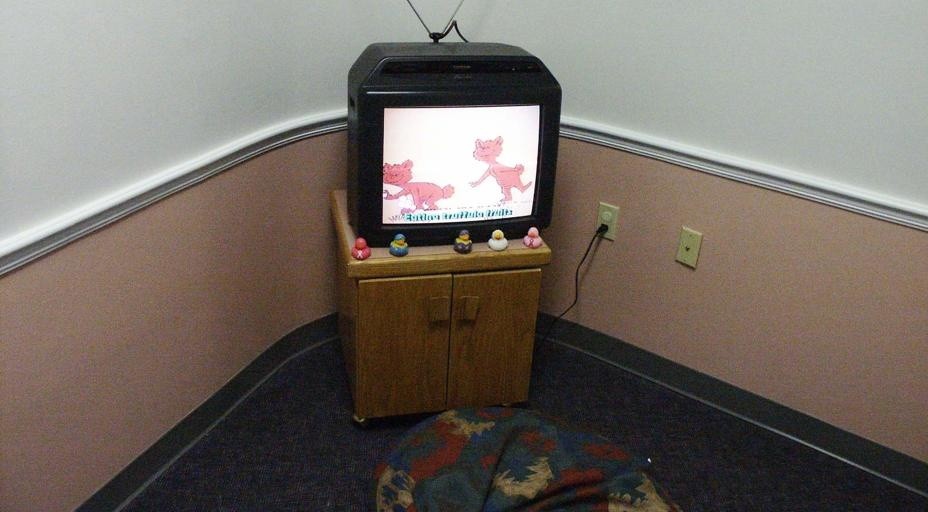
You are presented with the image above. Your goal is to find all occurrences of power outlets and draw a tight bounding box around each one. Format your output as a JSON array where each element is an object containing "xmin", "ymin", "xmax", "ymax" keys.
[{"xmin": 595, "ymin": 201, "xmax": 620, "ymax": 242}]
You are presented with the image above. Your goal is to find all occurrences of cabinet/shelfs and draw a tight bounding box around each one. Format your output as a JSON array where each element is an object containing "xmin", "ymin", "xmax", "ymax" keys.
[{"xmin": 329, "ymin": 189, "xmax": 552, "ymax": 426}]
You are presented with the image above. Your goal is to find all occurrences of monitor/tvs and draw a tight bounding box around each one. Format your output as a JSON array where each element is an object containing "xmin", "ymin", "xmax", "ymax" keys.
[{"xmin": 347, "ymin": 42, "xmax": 562, "ymax": 248}]
